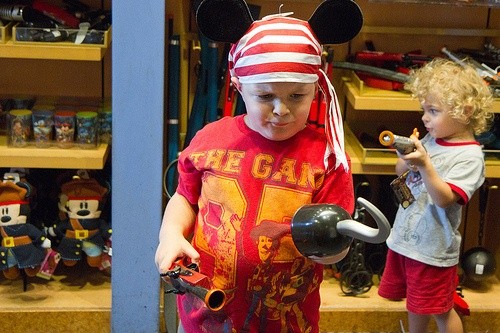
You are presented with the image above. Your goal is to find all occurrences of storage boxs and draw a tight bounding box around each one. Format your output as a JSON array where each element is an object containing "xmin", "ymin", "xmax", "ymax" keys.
[
  {"xmin": 344, "ymin": 121, "xmax": 399, "ymax": 165},
  {"xmin": 12, "ymin": 23, "xmax": 112, "ymax": 48}
]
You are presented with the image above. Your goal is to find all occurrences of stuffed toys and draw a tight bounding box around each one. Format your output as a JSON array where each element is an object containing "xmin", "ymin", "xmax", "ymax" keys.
[{"xmin": 0, "ymin": 173, "xmax": 110, "ymax": 279}]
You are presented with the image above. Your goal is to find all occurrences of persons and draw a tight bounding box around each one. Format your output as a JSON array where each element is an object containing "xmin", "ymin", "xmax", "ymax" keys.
[
  {"xmin": 377, "ymin": 61, "xmax": 493, "ymax": 333},
  {"xmin": 155, "ymin": 0, "xmax": 363, "ymax": 333}
]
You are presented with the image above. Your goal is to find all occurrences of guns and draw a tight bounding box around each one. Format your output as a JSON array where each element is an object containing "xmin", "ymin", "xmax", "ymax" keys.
[
  {"xmin": 439, "ymin": 45, "xmax": 500, "ymax": 83},
  {"xmin": 378, "ymin": 128, "xmax": 427, "ymax": 172},
  {"xmin": 159, "ymin": 257, "xmax": 226, "ymax": 311}
]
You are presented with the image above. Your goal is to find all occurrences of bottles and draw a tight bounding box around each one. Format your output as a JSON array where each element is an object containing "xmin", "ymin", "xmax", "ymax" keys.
[{"xmin": 0, "ymin": 94, "xmax": 112, "ymax": 149}]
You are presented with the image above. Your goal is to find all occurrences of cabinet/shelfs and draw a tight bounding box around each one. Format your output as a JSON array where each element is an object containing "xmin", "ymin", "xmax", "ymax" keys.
[
  {"xmin": 0, "ymin": 40, "xmax": 112, "ymax": 312},
  {"xmin": 319, "ymin": 75, "xmax": 500, "ymax": 313}
]
